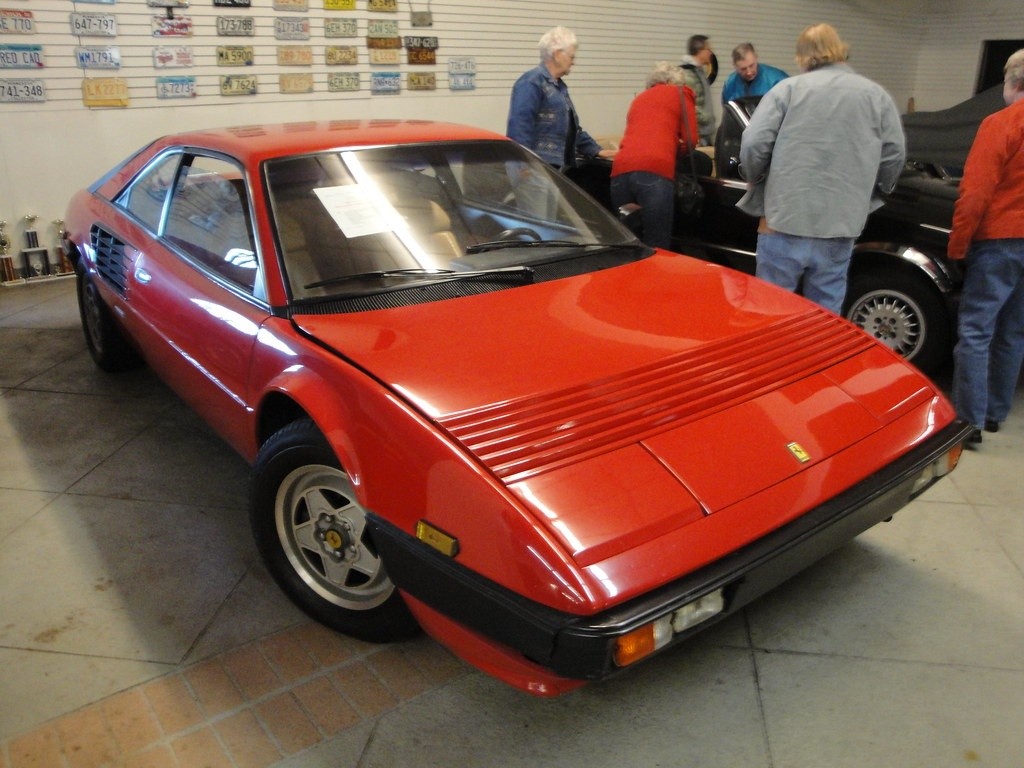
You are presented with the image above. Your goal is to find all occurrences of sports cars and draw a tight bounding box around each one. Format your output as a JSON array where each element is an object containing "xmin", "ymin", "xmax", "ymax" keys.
[{"xmin": 566, "ymin": 94, "xmax": 989, "ymax": 374}]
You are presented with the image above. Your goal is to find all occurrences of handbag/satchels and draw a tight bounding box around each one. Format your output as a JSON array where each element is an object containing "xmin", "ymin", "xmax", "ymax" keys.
[{"xmin": 673, "ymin": 168, "xmax": 704, "ymax": 217}]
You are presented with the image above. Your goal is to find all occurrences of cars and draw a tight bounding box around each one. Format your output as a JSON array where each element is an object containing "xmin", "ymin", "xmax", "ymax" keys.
[
  {"xmin": 894, "ymin": 85, "xmax": 1005, "ymax": 185},
  {"xmin": 61, "ymin": 116, "xmax": 974, "ymax": 698}
]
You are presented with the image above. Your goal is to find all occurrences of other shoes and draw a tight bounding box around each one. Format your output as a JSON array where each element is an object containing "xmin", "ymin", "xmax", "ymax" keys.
[
  {"xmin": 986, "ymin": 420, "xmax": 998, "ymax": 430},
  {"xmin": 968, "ymin": 429, "xmax": 983, "ymax": 442}
]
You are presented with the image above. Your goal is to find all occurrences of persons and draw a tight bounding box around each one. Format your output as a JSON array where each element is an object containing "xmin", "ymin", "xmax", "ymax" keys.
[
  {"xmin": 944, "ymin": 48, "xmax": 1024, "ymax": 444},
  {"xmin": 504, "ymin": 24, "xmax": 619, "ymax": 224},
  {"xmin": 610, "ymin": 62, "xmax": 700, "ymax": 251},
  {"xmin": 675, "ymin": 34, "xmax": 717, "ymax": 148},
  {"xmin": 735, "ymin": 22, "xmax": 907, "ymax": 317},
  {"xmin": 721, "ymin": 42, "xmax": 791, "ymax": 106}
]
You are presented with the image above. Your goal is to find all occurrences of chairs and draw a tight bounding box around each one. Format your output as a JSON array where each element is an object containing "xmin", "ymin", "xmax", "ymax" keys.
[{"xmin": 216, "ymin": 168, "xmax": 464, "ymax": 306}]
